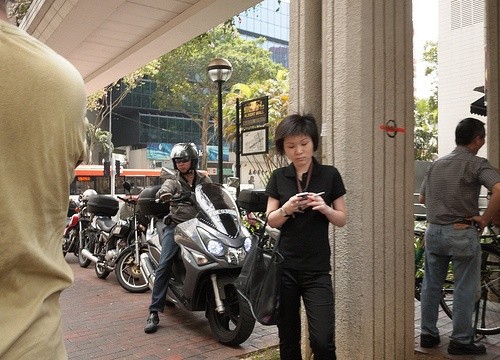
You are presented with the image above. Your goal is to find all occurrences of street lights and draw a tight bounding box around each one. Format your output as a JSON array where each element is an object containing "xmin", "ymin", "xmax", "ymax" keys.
[{"xmin": 207, "ymin": 58, "xmax": 233, "ymax": 186}]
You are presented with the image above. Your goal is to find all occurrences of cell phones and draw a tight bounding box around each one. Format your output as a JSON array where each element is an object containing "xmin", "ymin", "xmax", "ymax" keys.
[{"xmin": 296, "ymin": 192, "xmax": 308, "ymax": 201}]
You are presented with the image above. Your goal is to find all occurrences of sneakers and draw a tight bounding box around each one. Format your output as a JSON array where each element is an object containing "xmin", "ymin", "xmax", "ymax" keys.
[
  {"xmin": 144, "ymin": 313, "xmax": 159, "ymax": 333},
  {"xmin": 420, "ymin": 334, "xmax": 440, "ymax": 348},
  {"xmin": 447, "ymin": 340, "xmax": 486, "ymax": 355}
]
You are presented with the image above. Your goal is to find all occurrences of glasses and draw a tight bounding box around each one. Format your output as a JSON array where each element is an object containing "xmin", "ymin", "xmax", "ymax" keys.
[{"xmin": 174, "ymin": 157, "xmax": 190, "ymax": 162}]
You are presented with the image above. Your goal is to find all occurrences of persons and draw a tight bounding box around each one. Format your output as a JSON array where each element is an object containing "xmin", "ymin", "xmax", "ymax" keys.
[
  {"xmin": 265, "ymin": 113, "xmax": 348, "ymax": 360},
  {"xmin": 144, "ymin": 142, "xmax": 213, "ymax": 333},
  {"xmin": 0, "ymin": 0, "xmax": 88, "ymax": 360},
  {"xmin": 419, "ymin": 118, "xmax": 500, "ymax": 354}
]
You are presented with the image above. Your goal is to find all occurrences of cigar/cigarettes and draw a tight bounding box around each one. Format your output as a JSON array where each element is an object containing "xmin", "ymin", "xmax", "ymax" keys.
[{"xmin": 313, "ymin": 192, "xmax": 326, "ymax": 197}]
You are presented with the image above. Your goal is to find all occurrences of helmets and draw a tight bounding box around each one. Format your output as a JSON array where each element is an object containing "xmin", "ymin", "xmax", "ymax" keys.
[{"xmin": 171, "ymin": 143, "xmax": 199, "ymax": 159}]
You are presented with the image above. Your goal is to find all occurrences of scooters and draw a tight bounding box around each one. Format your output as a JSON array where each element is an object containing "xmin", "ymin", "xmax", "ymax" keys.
[{"xmin": 138, "ymin": 182, "xmax": 257, "ymax": 346}]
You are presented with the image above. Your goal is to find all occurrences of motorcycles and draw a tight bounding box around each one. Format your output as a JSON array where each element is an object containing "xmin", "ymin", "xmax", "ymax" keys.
[
  {"xmin": 60, "ymin": 188, "xmax": 99, "ymax": 269},
  {"xmin": 82, "ymin": 181, "xmax": 158, "ymax": 294}
]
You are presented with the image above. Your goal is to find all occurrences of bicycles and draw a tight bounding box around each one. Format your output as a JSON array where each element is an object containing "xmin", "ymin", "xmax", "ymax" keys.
[{"xmin": 411, "ymin": 213, "xmax": 500, "ymax": 343}]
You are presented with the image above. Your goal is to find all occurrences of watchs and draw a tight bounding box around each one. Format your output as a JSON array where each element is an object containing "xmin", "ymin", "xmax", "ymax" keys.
[{"xmin": 279, "ymin": 205, "xmax": 295, "ymax": 219}]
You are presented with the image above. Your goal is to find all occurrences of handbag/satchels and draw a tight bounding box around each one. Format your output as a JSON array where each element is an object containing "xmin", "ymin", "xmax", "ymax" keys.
[{"xmin": 234, "ymin": 210, "xmax": 285, "ymax": 325}]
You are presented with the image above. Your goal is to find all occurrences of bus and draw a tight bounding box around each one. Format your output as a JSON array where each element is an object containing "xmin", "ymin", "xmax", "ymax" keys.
[{"xmin": 69, "ymin": 165, "xmax": 213, "ymax": 206}]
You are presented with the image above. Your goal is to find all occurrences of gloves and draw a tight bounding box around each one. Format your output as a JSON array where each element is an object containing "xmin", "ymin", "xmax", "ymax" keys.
[{"xmin": 160, "ymin": 193, "xmax": 172, "ymax": 202}]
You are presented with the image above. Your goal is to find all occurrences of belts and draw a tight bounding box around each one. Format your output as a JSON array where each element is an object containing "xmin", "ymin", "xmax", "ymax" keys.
[{"xmin": 454, "ymin": 220, "xmax": 476, "ymax": 225}]
